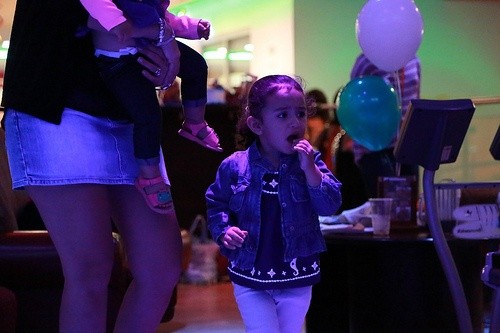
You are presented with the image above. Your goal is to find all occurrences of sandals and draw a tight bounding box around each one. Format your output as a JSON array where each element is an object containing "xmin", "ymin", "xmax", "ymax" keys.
[
  {"xmin": 137, "ymin": 174, "xmax": 175, "ymax": 214},
  {"xmin": 178, "ymin": 119, "xmax": 223, "ymax": 152}
]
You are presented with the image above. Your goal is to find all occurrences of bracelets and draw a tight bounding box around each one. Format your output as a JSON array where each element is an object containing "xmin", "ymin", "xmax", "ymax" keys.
[
  {"xmin": 158, "ymin": 18, "xmax": 164, "ymax": 43},
  {"xmin": 152, "ymin": 34, "xmax": 175, "ymax": 47}
]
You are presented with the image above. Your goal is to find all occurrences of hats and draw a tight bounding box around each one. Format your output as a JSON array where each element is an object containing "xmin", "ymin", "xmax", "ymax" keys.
[{"xmin": 305, "ymin": 89, "xmax": 330, "ymax": 120}]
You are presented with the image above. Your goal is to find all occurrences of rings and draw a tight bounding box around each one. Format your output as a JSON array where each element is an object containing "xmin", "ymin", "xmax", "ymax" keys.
[{"xmin": 155, "ymin": 68, "xmax": 161, "ymax": 76}]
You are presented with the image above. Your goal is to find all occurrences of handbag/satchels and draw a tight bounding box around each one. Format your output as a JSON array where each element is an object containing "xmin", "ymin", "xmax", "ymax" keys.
[{"xmin": 180, "ymin": 215, "xmax": 219, "ymax": 285}]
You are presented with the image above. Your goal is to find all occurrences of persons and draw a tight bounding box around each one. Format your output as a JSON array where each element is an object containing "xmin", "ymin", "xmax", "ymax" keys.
[
  {"xmin": 304, "ymin": 90, "xmax": 330, "ymax": 151},
  {"xmin": 76, "ymin": 0, "xmax": 221, "ymax": 214},
  {"xmin": 340, "ymin": 53, "xmax": 421, "ymax": 210},
  {"xmin": 205, "ymin": 75, "xmax": 341, "ymax": 333},
  {"xmin": 1, "ymin": 0, "xmax": 185, "ymax": 333}
]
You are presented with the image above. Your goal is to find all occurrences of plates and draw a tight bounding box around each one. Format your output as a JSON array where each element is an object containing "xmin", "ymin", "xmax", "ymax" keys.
[{"xmin": 321, "ymin": 224, "xmax": 373, "ymax": 234}]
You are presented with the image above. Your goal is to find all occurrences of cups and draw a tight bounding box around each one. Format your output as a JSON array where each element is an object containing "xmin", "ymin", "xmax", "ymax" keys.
[{"xmin": 369, "ymin": 198, "xmax": 393, "ymax": 236}]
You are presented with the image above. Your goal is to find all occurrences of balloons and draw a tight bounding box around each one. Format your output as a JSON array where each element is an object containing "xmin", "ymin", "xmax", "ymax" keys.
[
  {"xmin": 336, "ymin": 75, "xmax": 400, "ymax": 152},
  {"xmin": 355, "ymin": 0, "xmax": 423, "ymax": 72}
]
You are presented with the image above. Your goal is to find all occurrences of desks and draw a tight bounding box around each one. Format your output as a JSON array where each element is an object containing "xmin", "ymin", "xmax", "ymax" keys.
[{"xmin": 322, "ymin": 219, "xmax": 500, "ymax": 333}]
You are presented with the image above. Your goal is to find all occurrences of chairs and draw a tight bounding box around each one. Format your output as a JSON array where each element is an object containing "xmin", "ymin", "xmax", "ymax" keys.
[{"xmin": 0, "ymin": 126, "xmax": 122, "ymax": 333}]
